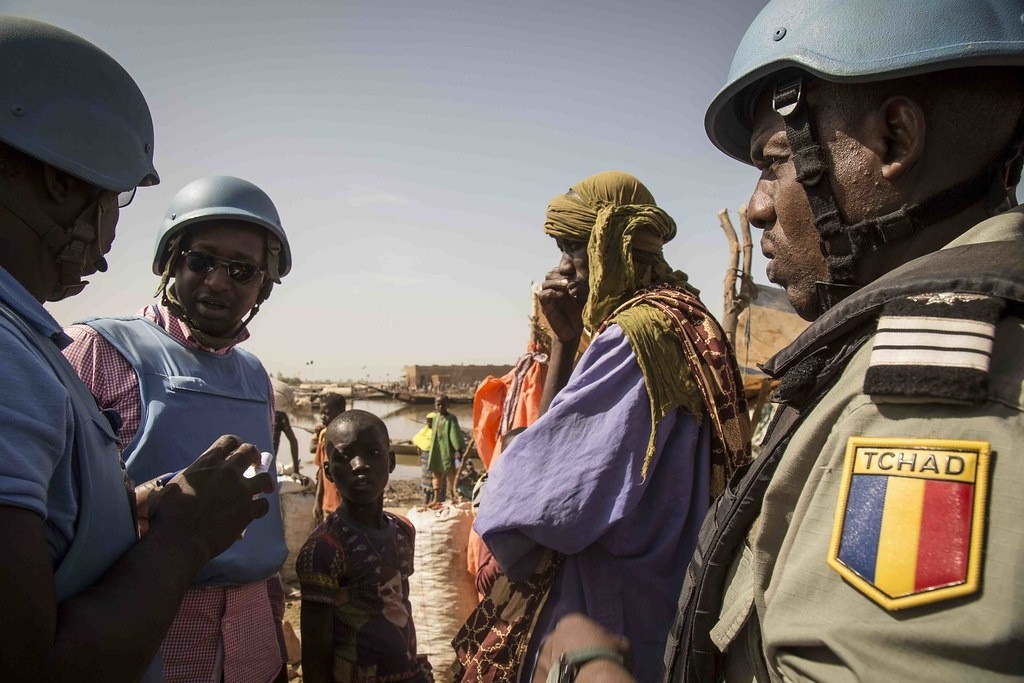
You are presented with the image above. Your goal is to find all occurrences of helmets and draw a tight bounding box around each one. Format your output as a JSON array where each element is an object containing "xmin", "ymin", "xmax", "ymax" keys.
[
  {"xmin": 0, "ymin": 13, "xmax": 160, "ymax": 192},
  {"xmin": 704, "ymin": 0, "xmax": 1024, "ymax": 168},
  {"xmin": 153, "ymin": 175, "xmax": 292, "ymax": 278}
]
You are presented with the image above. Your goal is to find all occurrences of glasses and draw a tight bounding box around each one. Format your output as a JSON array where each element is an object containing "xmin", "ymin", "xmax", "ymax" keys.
[
  {"xmin": 179, "ymin": 245, "xmax": 267, "ymax": 284},
  {"xmin": 118, "ymin": 186, "xmax": 137, "ymax": 208}
]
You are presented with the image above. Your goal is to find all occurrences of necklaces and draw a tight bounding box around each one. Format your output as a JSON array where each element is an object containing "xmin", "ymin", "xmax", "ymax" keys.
[{"xmin": 360, "ymin": 517, "xmax": 399, "ymax": 566}]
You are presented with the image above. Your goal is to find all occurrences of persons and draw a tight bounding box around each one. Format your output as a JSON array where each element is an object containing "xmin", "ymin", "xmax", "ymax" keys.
[
  {"xmin": 465, "ymin": 346, "xmax": 552, "ymax": 604},
  {"xmin": 452, "ymin": 172, "xmax": 752, "ymax": 683},
  {"xmin": 531, "ymin": 0, "xmax": 1024, "ymax": 683},
  {"xmin": 413, "ymin": 412, "xmax": 440, "ymax": 506},
  {"xmin": 63, "ymin": 173, "xmax": 294, "ymax": 683},
  {"xmin": 295, "ymin": 408, "xmax": 435, "ymax": 683},
  {"xmin": 426, "ymin": 395, "xmax": 462, "ymax": 510},
  {"xmin": 309, "ymin": 393, "xmax": 346, "ymax": 521},
  {"xmin": 0, "ymin": 17, "xmax": 272, "ymax": 683}
]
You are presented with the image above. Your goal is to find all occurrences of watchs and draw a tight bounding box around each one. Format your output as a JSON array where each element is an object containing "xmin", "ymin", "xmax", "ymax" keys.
[{"xmin": 547, "ymin": 648, "xmax": 622, "ymax": 683}]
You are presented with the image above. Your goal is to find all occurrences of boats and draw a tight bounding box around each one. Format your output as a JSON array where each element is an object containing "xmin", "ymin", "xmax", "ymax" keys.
[
  {"xmin": 292, "ymin": 425, "xmax": 480, "ymax": 457},
  {"xmin": 410, "ymin": 419, "xmax": 475, "ymax": 440},
  {"xmin": 359, "ymin": 381, "xmax": 474, "ymax": 404}
]
